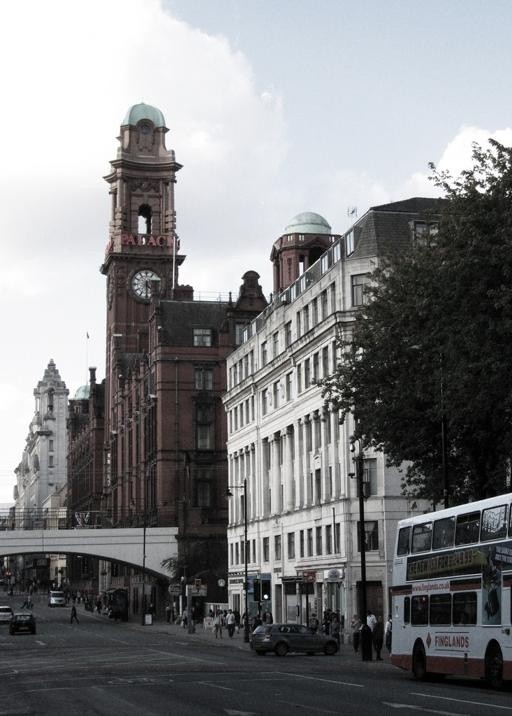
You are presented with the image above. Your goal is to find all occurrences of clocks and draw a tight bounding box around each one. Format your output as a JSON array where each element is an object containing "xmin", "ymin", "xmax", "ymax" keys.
[{"xmin": 126, "ymin": 264, "xmax": 167, "ymax": 303}]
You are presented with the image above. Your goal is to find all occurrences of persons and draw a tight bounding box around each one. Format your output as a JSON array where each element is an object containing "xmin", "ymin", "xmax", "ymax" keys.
[
  {"xmin": 209, "ymin": 608, "xmax": 273, "ymax": 639},
  {"xmin": 310, "ymin": 609, "xmax": 392, "ymax": 661},
  {"xmin": 165, "ymin": 602, "xmax": 196, "ymax": 629},
  {"xmin": 6, "ymin": 579, "xmax": 102, "ymax": 625}
]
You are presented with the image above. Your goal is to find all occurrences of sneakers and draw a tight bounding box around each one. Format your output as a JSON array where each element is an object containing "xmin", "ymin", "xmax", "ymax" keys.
[{"xmin": 376, "ymin": 657, "xmax": 385, "ymax": 661}]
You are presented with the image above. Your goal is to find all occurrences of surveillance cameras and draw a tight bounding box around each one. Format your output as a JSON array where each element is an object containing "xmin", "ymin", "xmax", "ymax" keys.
[{"xmin": 348, "ymin": 473, "xmax": 355, "ymax": 476}]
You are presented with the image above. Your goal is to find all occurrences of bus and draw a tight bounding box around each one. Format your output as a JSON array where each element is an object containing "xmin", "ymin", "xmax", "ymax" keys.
[{"xmin": 387, "ymin": 489, "xmax": 512, "ymax": 690}]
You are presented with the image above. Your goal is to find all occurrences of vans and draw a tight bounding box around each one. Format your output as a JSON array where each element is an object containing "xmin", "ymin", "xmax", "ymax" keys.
[{"xmin": 47, "ymin": 589, "xmax": 67, "ymax": 607}]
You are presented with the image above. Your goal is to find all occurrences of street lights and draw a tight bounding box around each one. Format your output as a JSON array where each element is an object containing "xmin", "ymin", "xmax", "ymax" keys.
[
  {"xmin": 222, "ymin": 477, "xmax": 250, "ymax": 642},
  {"xmin": 129, "ymin": 495, "xmax": 147, "ymax": 625}
]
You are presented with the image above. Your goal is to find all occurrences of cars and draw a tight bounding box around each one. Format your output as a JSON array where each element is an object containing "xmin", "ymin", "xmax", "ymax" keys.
[
  {"xmin": 0, "ymin": 604, "xmax": 15, "ymax": 623},
  {"xmin": 248, "ymin": 621, "xmax": 340, "ymax": 657},
  {"xmin": 7, "ymin": 611, "xmax": 38, "ymax": 635}
]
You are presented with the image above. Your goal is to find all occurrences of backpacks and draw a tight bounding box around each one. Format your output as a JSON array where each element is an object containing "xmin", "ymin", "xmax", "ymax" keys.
[{"xmin": 310, "ymin": 619, "xmax": 317, "ymax": 629}]
